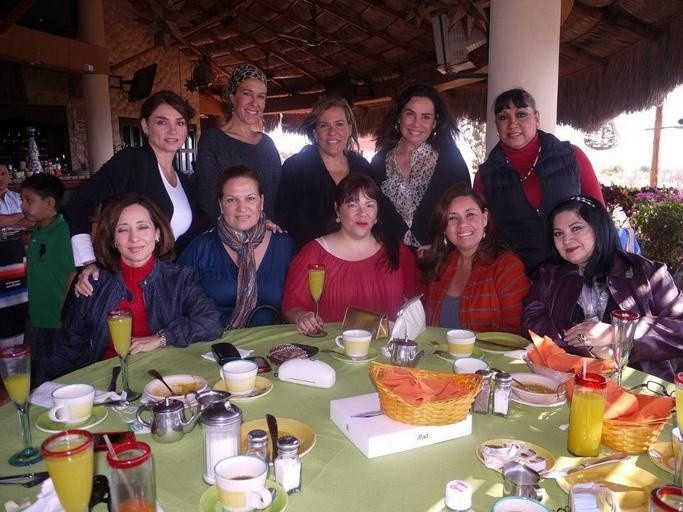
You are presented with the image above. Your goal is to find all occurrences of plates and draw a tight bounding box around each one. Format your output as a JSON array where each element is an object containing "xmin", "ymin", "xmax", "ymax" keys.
[
  {"xmin": 139, "ymin": 386, "xmax": 211, "ymax": 410},
  {"xmin": 474, "ymin": 331, "xmax": 531, "ymax": 354},
  {"xmin": 646, "ymin": 440, "xmax": 681, "ymax": 477},
  {"xmin": 212, "ymin": 375, "xmax": 274, "ymax": 403},
  {"xmin": 557, "ymin": 455, "xmax": 665, "ymax": 512},
  {"xmin": 329, "ymin": 346, "xmax": 383, "ymax": 366},
  {"xmin": 198, "ymin": 477, "xmax": 291, "ymax": 511},
  {"xmin": 433, "ymin": 342, "xmax": 486, "ymax": 364},
  {"xmin": 510, "ymin": 396, "xmax": 569, "ymax": 407},
  {"xmin": 236, "ymin": 415, "xmax": 319, "ymax": 468},
  {"xmin": 475, "ymin": 437, "xmax": 558, "ymax": 476},
  {"xmin": 34, "ymin": 405, "xmax": 110, "ymax": 434}
]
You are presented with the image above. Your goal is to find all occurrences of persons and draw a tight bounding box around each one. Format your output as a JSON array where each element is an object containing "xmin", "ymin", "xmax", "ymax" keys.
[
  {"xmin": 20, "ymin": 173, "xmax": 78, "ymax": 392},
  {"xmin": 276, "ymin": 97, "xmax": 378, "ymax": 237},
  {"xmin": 176, "ymin": 166, "xmax": 293, "ymax": 328},
  {"xmin": 371, "ymin": 83, "xmax": 471, "ymax": 274},
  {"xmin": 282, "ymin": 173, "xmax": 427, "ymax": 335},
  {"xmin": 424, "ymin": 184, "xmax": 530, "ymax": 335},
  {"xmin": 196, "ymin": 64, "xmax": 282, "ymax": 234},
  {"xmin": 473, "ymin": 89, "xmax": 606, "ymax": 280},
  {"xmin": 61, "ymin": 90, "xmax": 197, "ymax": 326},
  {"xmin": 521, "ymin": 196, "xmax": 683, "ymax": 384},
  {"xmin": 0, "ymin": 159, "xmax": 36, "ymax": 266},
  {"xmin": 50, "ymin": 193, "xmax": 223, "ymax": 381}
]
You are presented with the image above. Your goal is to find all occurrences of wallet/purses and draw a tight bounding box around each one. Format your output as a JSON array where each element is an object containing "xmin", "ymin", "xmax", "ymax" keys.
[{"xmin": 340, "ymin": 306, "xmax": 389, "ymax": 339}]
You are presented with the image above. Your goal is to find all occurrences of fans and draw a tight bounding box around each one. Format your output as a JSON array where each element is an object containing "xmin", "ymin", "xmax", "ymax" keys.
[{"xmin": 276, "ymin": 0, "xmax": 358, "ymax": 54}]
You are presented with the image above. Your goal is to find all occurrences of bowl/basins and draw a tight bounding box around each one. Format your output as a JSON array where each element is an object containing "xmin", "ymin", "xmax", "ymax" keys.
[
  {"xmin": 521, "ymin": 351, "xmax": 576, "ymax": 383},
  {"xmin": 452, "ymin": 357, "xmax": 492, "ymax": 374},
  {"xmin": 143, "ymin": 374, "xmax": 208, "ymax": 405},
  {"xmin": 508, "ymin": 376, "xmax": 572, "ymax": 405}
]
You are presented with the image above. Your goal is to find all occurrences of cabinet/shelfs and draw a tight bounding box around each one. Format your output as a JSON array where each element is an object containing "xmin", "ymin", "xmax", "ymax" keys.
[{"xmin": 0, "ymin": 105, "xmax": 74, "ymax": 176}]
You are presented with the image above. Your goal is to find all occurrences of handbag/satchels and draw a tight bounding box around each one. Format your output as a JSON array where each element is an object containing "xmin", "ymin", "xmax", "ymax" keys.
[{"xmin": 392, "ymin": 291, "xmax": 427, "ymax": 342}]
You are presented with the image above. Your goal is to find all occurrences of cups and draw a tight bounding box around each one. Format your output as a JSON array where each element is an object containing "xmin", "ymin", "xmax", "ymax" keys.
[
  {"xmin": 213, "ymin": 454, "xmax": 276, "ymax": 511},
  {"xmin": 105, "ymin": 305, "xmax": 141, "ymax": 403},
  {"xmin": 490, "ymin": 494, "xmax": 554, "ymax": 512},
  {"xmin": 48, "ymin": 383, "xmax": 96, "ymax": 426},
  {"xmin": 565, "ymin": 374, "xmax": 607, "ymax": 458},
  {"xmin": 218, "ymin": 359, "xmax": 259, "ymax": 396},
  {"xmin": 334, "ymin": 328, "xmax": 373, "ymax": 358},
  {"xmin": 307, "ymin": 262, "xmax": 329, "ymax": 338},
  {"xmin": 104, "ymin": 439, "xmax": 157, "ymax": 512},
  {"xmin": 646, "ymin": 483, "xmax": 683, "ymax": 512},
  {"xmin": 0, "ymin": 343, "xmax": 43, "ymax": 467},
  {"xmin": 672, "ymin": 368, "xmax": 683, "ymax": 441},
  {"xmin": 39, "ymin": 428, "xmax": 96, "ymax": 512},
  {"xmin": 446, "ymin": 329, "xmax": 476, "ymax": 359},
  {"xmin": 610, "ymin": 308, "xmax": 640, "ymax": 388}
]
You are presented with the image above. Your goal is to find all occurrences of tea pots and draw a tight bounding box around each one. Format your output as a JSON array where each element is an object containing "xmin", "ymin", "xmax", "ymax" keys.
[{"xmin": 386, "ymin": 334, "xmax": 426, "ymax": 369}]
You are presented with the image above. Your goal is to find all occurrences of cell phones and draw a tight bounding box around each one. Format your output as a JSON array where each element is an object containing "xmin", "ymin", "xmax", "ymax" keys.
[
  {"xmin": 95, "ymin": 432, "xmax": 136, "ymax": 451},
  {"xmin": 212, "ymin": 342, "xmax": 241, "ymax": 366},
  {"xmin": 244, "ymin": 356, "xmax": 272, "ymax": 373}
]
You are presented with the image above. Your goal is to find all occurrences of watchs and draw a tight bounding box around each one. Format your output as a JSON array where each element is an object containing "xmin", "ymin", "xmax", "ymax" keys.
[{"xmin": 156, "ymin": 330, "xmax": 167, "ymax": 347}]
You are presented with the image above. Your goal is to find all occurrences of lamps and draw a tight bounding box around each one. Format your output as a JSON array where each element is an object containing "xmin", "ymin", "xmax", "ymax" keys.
[
  {"xmin": 109, "ymin": 63, "xmax": 158, "ymax": 101},
  {"xmin": 431, "ymin": 12, "xmax": 489, "ymax": 76},
  {"xmin": 324, "ymin": 70, "xmax": 376, "ymax": 109}
]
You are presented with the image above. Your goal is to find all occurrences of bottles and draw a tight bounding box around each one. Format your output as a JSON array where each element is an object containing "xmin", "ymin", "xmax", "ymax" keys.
[
  {"xmin": 62, "ymin": 154, "xmax": 69, "ymax": 174},
  {"xmin": 201, "ymin": 403, "xmax": 243, "ymax": 487},
  {"xmin": 246, "ymin": 429, "xmax": 269, "ymax": 479},
  {"xmin": 472, "ymin": 370, "xmax": 492, "ymax": 415},
  {"xmin": 492, "ymin": 372, "xmax": 513, "ymax": 417},
  {"xmin": 55, "ymin": 157, "xmax": 62, "ymax": 175},
  {"xmin": 41, "ymin": 160, "xmax": 54, "ymax": 175},
  {"xmin": 274, "ymin": 436, "xmax": 302, "ymax": 497}
]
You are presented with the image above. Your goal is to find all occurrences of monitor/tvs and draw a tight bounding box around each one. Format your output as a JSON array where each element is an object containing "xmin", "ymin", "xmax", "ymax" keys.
[
  {"xmin": 128, "ymin": 63, "xmax": 158, "ymax": 102},
  {"xmin": 324, "ymin": 70, "xmax": 354, "ymax": 111}
]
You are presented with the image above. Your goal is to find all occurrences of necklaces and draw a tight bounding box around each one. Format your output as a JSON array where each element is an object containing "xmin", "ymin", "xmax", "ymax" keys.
[{"xmin": 505, "ymin": 146, "xmax": 541, "ymax": 182}]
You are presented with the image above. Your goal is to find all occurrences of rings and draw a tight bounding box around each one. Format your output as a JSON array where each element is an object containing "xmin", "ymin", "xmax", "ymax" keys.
[{"xmin": 576, "ymin": 334, "xmax": 587, "ymax": 343}]
